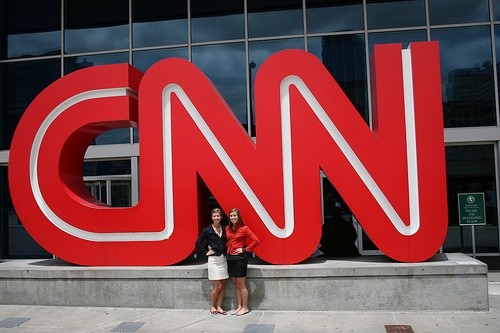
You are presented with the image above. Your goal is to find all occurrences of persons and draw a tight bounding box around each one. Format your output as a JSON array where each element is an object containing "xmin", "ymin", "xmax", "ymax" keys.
[
  {"xmin": 208, "ymin": 207, "xmax": 260, "ymax": 315},
  {"xmin": 321, "ymin": 207, "xmax": 360, "ymax": 258},
  {"xmin": 196, "ymin": 207, "xmax": 229, "ymax": 314}
]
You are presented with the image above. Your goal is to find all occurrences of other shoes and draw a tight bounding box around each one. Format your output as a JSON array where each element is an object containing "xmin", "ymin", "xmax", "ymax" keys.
[
  {"xmin": 219, "ymin": 310, "xmax": 227, "ymax": 315},
  {"xmin": 210, "ymin": 309, "xmax": 218, "ymax": 314},
  {"xmin": 237, "ymin": 309, "xmax": 249, "ymax": 316},
  {"xmin": 232, "ymin": 309, "xmax": 240, "ymax": 315}
]
